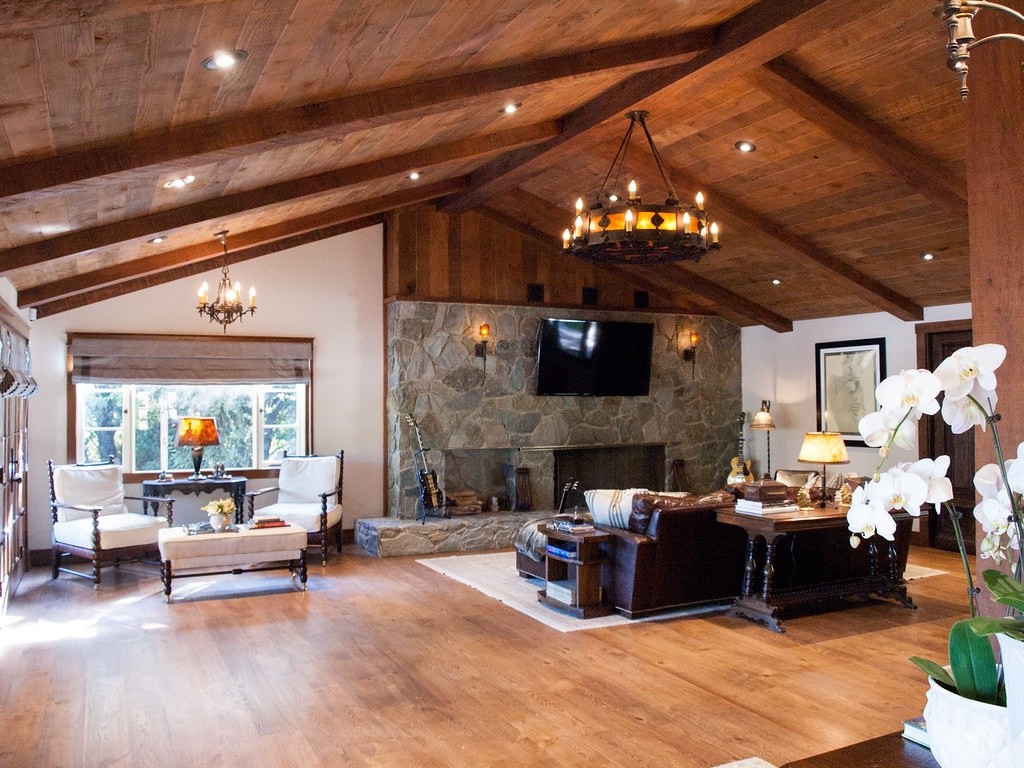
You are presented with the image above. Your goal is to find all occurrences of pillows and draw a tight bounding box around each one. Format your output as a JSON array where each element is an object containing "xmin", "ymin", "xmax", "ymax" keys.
[
  {"xmin": 804, "ymin": 473, "xmax": 855, "ymax": 490},
  {"xmin": 585, "ymin": 488, "xmax": 690, "ymax": 531}
]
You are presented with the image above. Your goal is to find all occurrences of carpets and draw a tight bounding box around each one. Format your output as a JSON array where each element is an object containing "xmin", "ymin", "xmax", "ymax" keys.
[{"xmin": 415, "ymin": 550, "xmax": 949, "ymax": 634}]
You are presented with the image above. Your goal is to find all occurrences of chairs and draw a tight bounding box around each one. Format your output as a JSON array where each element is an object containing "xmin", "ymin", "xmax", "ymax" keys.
[
  {"xmin": 48, "ymin": 454, "xmax": 175, "ymax": 591},
  {"xmin": 244, "ymin": 448, "xmax": 344, "ymax": 567}
]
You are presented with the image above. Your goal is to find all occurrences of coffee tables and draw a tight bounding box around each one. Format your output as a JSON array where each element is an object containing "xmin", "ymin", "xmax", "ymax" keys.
[{"xmin": 159, "ymin": 521, "xmax": 307, "ymax": 602}]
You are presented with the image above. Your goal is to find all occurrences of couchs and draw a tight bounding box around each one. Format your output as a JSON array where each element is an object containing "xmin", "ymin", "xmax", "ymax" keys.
[{"xmin": 514, "ymin": 476, "xmax": 872, "ymax": 620}]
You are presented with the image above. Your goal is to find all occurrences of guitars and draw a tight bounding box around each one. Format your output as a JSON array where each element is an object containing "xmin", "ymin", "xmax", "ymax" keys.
[
  {"xmin": 404, "ymin": 412, "xmax": 443, "ymax": 509},
  {"xmin": 557, "ymin": 477, "xmax": 579, "ymax": 514},
  {"xmin": 727, "ymin": 412, "xmax": 755, "ymax": 485}
]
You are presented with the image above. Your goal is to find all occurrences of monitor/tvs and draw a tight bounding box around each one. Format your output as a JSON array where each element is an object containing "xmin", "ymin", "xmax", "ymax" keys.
[{"xmin": 536, "ymin": 318, "xmax": 654, "ymax": 396}]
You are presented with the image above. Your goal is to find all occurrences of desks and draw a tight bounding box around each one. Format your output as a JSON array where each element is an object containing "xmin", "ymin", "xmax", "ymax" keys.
[
  {"xmin": 715, "ymin": 505, "xmax": 935, "ymax": 633},
  {"xmin": 780, "ymin": 731, "xmax": 940, "ymax": 768},
  {"xmin": 142, "ymin": 476, "xmax": 247, "ymax": 562}
]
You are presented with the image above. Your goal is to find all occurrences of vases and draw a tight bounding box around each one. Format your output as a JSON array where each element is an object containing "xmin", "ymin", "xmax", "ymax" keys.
[{"xmin": 923, "ymin": 618, "xmax": 1024, "ymax": 768}]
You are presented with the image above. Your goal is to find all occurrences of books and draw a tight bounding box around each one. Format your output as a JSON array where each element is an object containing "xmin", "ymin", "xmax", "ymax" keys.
[
  {"xmin": 734, "ymin": 498, "xmax": 799, "ymax": 514},
  {"xmin": 246, "ymin": 516, "xmax": 290, "ymax": 530},
  {"xmin": 546, "ymin": 544, "xmax": 576, "ymax": 559}
]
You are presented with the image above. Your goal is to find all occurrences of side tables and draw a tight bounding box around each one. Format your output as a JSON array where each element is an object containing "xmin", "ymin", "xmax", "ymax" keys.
[{"xmin": 538, "ymin": 525, "xmax": 612, "ymax": 619}]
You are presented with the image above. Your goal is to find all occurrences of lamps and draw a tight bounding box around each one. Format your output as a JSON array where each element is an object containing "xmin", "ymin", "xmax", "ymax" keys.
[
  {"xmin": 750, "ymin": 400, "xmax": 775, "ymax": 478},
  {"xmin": 196, "ymin": 229, "xmax": 257, "ymax": 334},
  {"xmin": 173, "ymin": 411, "xmax": 220, "ymax": 481},
  {"xmin": 682, "ymin": 330, "xmax": 700, "ymax": 380},
  {"xmin": 797, "ymin": 430, "xmax": 850, "ymax": 507},
  {"xmin": 475, "ymin": 324, "xmax": 491, "ymax": 384},
  {"xmin": 563, "ymin": 109, "xmax": 720, "ymax": 267}
]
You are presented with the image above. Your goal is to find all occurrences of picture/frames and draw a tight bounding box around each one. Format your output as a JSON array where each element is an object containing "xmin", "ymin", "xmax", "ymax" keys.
[{"xmin": 815, "ymin": 336, "xmax": 887, "ymax": 449}]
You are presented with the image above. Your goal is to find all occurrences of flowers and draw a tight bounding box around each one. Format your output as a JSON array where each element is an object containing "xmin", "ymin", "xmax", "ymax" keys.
[{"xmin": 847, "ymin": 341, "xmax": 1024, "ymax": 702}]
